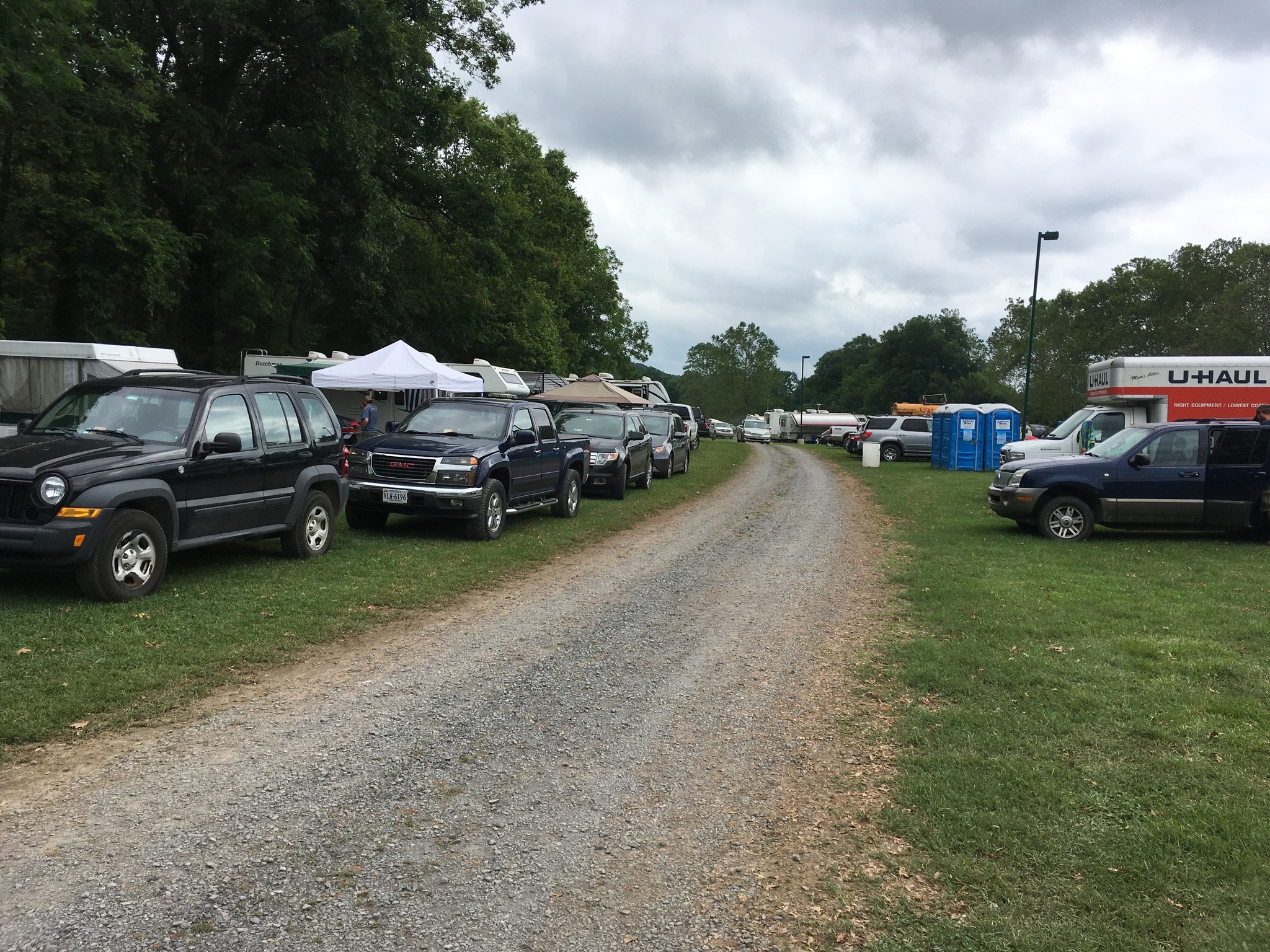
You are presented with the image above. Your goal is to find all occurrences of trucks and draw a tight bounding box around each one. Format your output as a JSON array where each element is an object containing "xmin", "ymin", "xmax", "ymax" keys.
[
  {"xmin": 1020, "ymin": 423, "xmax": 1051, "ymax": 441},
  {"xmin": 239, "ymin": 348, "xmax": 532, "ymax": 452},
  {"xmin": 746, "ymin": 393, "xmax": 951, "ymax": 455},
  {"xmin": 0, "ymin": 339, "xmax": 195, "ymax": 438},
  {"xmin": 513, "ymin": 369, "xmax": 671, "ymax": 418},
  {"xmin": 998, "ymin": 356, "xmax": 1270, "ymax": 462}
]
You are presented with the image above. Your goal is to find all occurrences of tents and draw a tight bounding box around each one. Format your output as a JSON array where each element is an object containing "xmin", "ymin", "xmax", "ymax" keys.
[{"xmin": 271, "ymin": 340, "xmax": 483, "ymax": 398}]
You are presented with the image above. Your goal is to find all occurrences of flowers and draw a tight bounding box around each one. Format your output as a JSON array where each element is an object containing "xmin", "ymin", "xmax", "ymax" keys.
[{"xmin": 349, "ymin": 421, "xmax": 359, "ymax": 427}]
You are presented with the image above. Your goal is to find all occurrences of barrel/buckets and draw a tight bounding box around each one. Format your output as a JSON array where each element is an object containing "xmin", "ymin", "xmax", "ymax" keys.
[{"xmin": 862, "ymin": 443, "xmax": 880, "ymax": 468}]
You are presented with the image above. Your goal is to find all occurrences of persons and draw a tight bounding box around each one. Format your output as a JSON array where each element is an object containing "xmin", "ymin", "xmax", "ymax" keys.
[
  {"xmin": 1253, "ymin": 404, "xmax": 1270, "ymax": 543},
  {"xmin": 360, "ymin": 396, "xmax": 378, "ymax": 440}
]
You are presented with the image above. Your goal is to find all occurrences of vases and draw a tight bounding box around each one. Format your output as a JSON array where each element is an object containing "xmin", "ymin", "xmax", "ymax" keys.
[{"xmin": 352, "ymin": 425, "xmax": 356, "ymax": 429}]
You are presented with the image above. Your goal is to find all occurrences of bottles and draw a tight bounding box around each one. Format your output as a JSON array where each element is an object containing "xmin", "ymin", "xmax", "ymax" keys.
[{"xmin": 360, "ymin": 404, "xmax": 365, "ymax": 408}]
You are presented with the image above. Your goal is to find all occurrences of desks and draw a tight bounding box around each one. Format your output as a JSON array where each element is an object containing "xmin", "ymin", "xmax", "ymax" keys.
[{"xmin": 342, "ymin": 432, "xmax": 360, "ymax": 445}]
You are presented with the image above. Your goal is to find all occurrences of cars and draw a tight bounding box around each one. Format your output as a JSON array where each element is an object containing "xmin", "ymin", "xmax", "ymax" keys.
[
  {"xmin": 736, "ymin": 419, "xmax": 772, "ymax": 444},
  {"xmin": 690, "ymin": 406, "xmax": 737, "ymax": 440}
]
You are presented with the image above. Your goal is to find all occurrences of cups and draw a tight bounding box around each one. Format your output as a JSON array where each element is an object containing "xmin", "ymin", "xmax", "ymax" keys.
[{"xmin": 353, "ymin": 425, "xmax": 356, "ymax": 433}]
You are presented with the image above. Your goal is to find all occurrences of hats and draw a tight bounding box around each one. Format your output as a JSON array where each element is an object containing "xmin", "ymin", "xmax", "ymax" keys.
[
  {"xmin": 1253, "ymin": 404, "xmax": 1270, "ymax": 422},
  {"xmin": 363, "ymin": 396, "xmax": 372, "ymax": 401}
]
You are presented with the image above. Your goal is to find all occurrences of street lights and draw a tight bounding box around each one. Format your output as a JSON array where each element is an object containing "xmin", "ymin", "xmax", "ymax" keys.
[
  {"xmin": 800, "ymin": 355, "xmax": 810, "ymax": 439},
  {"xmin": 1021, "ymin": 231, "xmax": 1060, "ymax": 441}
]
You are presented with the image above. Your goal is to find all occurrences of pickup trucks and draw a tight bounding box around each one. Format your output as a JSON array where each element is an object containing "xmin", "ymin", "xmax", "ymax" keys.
[
  {"xmin": 646, "ymin": 403, "xmax": 706, "ymax": 450},
  {"xmin": 345, "ymin": 396, "xmax": 591, "ymax": 542}
]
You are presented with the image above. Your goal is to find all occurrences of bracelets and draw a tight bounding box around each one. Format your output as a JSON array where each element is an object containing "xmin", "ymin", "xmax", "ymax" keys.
[{"xmin": 360, "ymin": 428, "xmax": 363, "ymax": 431}]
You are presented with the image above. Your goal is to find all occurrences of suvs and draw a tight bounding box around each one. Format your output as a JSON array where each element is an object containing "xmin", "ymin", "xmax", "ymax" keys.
[
  {"xmin": 857, "ymin": 413, "xmax": 933, "ymax": 462},
  {"xmin": 621, "ymin": 407, "xmax": 691, "ymax": 479},
  {"xmin": 553, "ymin": 407, "xmax": 655, "ymax": 500},
  {"xmin": 1, "ymin": 368, "xmax": 350, "ymax": 602},
  {"xmin": 987, "ymin": 418, "xmax": 1270, "ymax": 545}
]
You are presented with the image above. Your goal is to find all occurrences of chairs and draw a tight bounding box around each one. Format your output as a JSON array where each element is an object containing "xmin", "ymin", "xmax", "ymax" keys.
[{"xmin": 1166, "ymin": 436, "xmax": 1187, "ymax": 465}]
